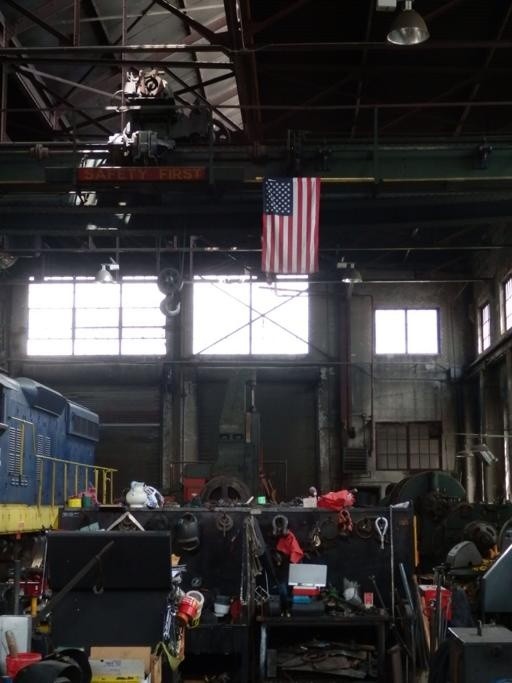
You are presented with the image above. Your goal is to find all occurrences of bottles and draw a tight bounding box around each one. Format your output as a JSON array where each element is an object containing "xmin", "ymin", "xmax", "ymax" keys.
[{"xmin": 126, "ymin": 482, "xmax": 147, "ymax": 507}]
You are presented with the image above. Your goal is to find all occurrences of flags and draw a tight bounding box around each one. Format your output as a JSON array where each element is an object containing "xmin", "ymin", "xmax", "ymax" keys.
[{"xmin": 261, "ymin": 176, "xmax": 321, "ymax": 274}]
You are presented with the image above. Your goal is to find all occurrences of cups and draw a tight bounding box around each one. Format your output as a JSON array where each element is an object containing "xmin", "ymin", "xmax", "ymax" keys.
[{"xmin": 5, "ymin": 653, "xmax": 41, "ymax": 681}]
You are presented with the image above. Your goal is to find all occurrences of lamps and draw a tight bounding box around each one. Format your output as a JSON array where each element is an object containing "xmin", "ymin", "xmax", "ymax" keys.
[{"xmin": 388, "ymin": 0, "xmax": 430, "ymax": 46}]
